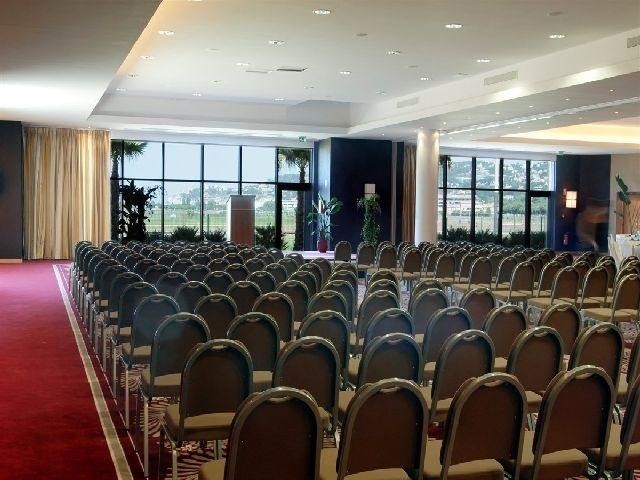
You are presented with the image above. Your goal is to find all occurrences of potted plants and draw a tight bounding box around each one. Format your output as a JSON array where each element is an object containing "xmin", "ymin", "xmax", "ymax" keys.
[{"xmin": 305, "ymin": 191, "xmax": 344, "ymax": 253}]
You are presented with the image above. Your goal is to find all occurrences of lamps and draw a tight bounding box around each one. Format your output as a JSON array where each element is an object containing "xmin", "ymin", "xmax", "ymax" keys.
[
  {"xmin": 562, "ymin": 190, "xmax": 578, "ymax": 208},
  {"xmin": 365, "ymin": 184, "xmax": 376, "ymax": 201}
]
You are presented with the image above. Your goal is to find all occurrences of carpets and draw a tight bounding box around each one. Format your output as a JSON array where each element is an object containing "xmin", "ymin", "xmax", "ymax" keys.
[{"xmin": 0, "ymin": 259, "xmax": 229, "ymax": 480}]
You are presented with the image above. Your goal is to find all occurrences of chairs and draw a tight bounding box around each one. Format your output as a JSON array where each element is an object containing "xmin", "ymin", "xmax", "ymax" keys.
[
  {"xmin": 224, "ymin": 262, "xmax": 251, "ymax": 282},
  {"xmin": 492, "ymin": 244, "xmax": 503, "ymax": 253},
  {"xmin": 420, "ymin": 252, "xmax": 456, "ymax": 285},
  {"xmin": 393, "ymin": 250, "xmax": 422, "ymax": 292},
  {"xmin": 470, "ymin": 245, "xmax": 484, "ymax": 253},
  {"xmin": 595, "ymin": 255, "xmax": 616, "ymax": 270},
  {"xmin": 252, "ymin": 293, "xmax": 295, "ymax": 342},
  {"xmin": 134, "ymin": 312, "xmax": 212, "ymax": 477},
  {"xmin": 577, "ymin": 373, "xmax": 639, "ymax": 479},
  {"xmin": 501, "ymin": 247, "xmax": 512, "ymax": 257},
  {"xmin": 116, "ymin": 294, "xmax": 181, "ymax": 430},
  {"xmin": 193, "ymin": 292, "xmax": 238, "ymax": 339},
  {"xmin": 421, "ymin": 307, "xmax": 474, "ymax": 380},
  {"xmin": 224, "ymin": 241, "xmax": 236, "ymax": 247},
  {"xmin": 123, "ymin": 254, "xmax": 145, "ymax": 268},
  {"xmin": 173, "ymin": 258, "xmax": 197, "ymax": 274},
  {"xmin": 366, "ymin": 246, "xmax": 398, "ymax": 288},
  {"xmin": 599, "ymin": 260, "xmax": 617, "ymax": 296},
  {"xmin": 582, "ymin": 273, "xmax": 640, "ymax": 323},
  {"xmin": 400, "ymin": 245, "xmax": 413, "ymax": 264},
  {"xmin": 156, "ymin": 270, "xmax": 190, "ymax": 297},
  {"xmin": 203, "ymin": 270, "xmax": 236, "ymax": 294},
  {"xmin": 313, "ymin": 257, "xmax": 332, "ymax": 284},
  {"xmin": 426, "ymin": 248, "xmax": 445, "ymax": 278},
  {"xmin": 279, "ymin": 280, "xmax": 309, "ymax": 322},
  {"xmin": 351, "ymin": 244, "xmax": 376, "ymax": 282},
  {"xmin": 616, "ymin": 331, "xmax": 640, "ymax": 404},
  {"xmin": 478, "ymin": 256, "xmax": 518, "ymax": 290},
  {"xmin": 224, "ymin": 246, "xmax": 240, "ymax": 255},
  {"xmin": 451, "ymin": 256, "xmax": 492, "ymax": 306},
  {"xmin": 542, "ymin": 247, "xmax": 557, "ymax": 261},
  {"xmin": 73, "ymin": 245, "xmax": 93, "ymax": 298},
  {"xmin": 492, "ymin": 261, "xmax": 535, "ymax": 312},
  {"xmin": 480, "ymin": 305, "xmax": 529, "ymax": 373},
  {"xmin": 588, "ymin": 265, "xmax": 640, "ymax": 308},
  {"xmin": 402, "ymin": 246, "xmax": 419, "ymax": 266},
  {"xmin": 363, "ymin": 278, "xmax": 400, "ymax": 304},
  {"xmin": 408, "ymin": 279, "xmax": 445, "ymax": 313},
  {"xmin": 454, "ymin": 252, "xmax": 479, "ymax": 283},
  {"xmin": 553, "ymin": 256, "xmax": 570, "ymax": 268},
  {"xmin": 368, "ymin": 270, "xmax": 399, "ymax": 286},
  {"xmin": 485, "ymin": 242, "xmax": 495, "ymax": 250},
  {"xmin": 419, "ymin": 329, "xmax": 496, "ymax": 427},
  {"xmin": 448, "ymin": 246, "xmax": 462, "ymax": 254},
  {"xmin": 184, "ymin": 264, "xmax": 212, "ymax": 286},
  {"xmin": 227, "ymin": 280, "xmax": 263, "ymax": 314},
  {"xmin": 465, "ymin": 243, "xmax": 476, "ymax": 251},
  {"xmin": 460, "ymin": 241, "xmax": 468, "ymax": 248},
  {"xmin": 133, "ymin": 244, "xmax": 147, "ymax": 254},
  {"xmin": 139, "ymin": 247, "xmax": 156, "ymax": 258},
  {"xmin": 422, "ymin": 243, "xmax": 436, "ymax": 257},
  {"xmin": 110, "ymin": 246, "xmax": 127, "ymax": 257},
  {"xmin": 329, "ymin": 240, "xmax": 352, "ymax": 267},
  {"xmin": 253, "ymin": 246, "xmax": 266, "ymax": 254},
  {"xmin": 157, "ymin": 254, "xmax": 180, "ymax": 268},
  {"xmin": 599, "ymin": 260, "xmax": 617, "ymax": 289},
  {"xmin": 178, "ymin": 249, "xmax": 195, "ymax": 255},
  {"xmin": 197, "ymin": 247, "xmax": 213, "ymax": 253},
  {"xmin": 238, "ymin": 244, "xmax": 251, "ymax": 250},
  {"xmin": 133, "ymin": 258, "xmax": 159, "ymax": 276},
  {"xmin": 186, "ymin": 244, "xmax": 202, "ymax": 252},
  {"xmin": 454, "ymin": 248, "xmax": 468, "ymax": 272},
  {"xmin": 398, "ymin": 241, "xmax": 413, "ymax": 268},
  {"xmin": 246, "ymin": 271, "xmax": 277, "ymax": 294},
  {"xmin": 581, "ymin": 265, "xmax": 640, "ymax": 331},
  {"xmin": 528, "ymin": 255, "xmax": 543, "ymax": 283},
  {"xmin": 526, "ymin": 266, "xmax": 581, "ymax": 325},
  {"xmin": 329, "ymin": 269, "xmax": 359, "ymax": 308},
  {"xmin": 476, "ymin": 247, "xmax": 490, "ymax": 257},
  {"xmin": 81, "ymin": 253, "xmax": 112, "ymax": 323},
  {"xmin": 158, "ymin": 338, "xmax": 253, "ymax": 477},
  {"xmin": 151, "ymin": 239, "xmax": 166, "ymax": 248},
  {"xmin": 459, "ymin": 252, "xmax": 479, "ymax": 283},
  {"xmin": 300, "ymin": 262, "xmax": 324, "ymax": 290},
  {"xmin": 423, "ymin": 246, "xmax": 440, "ymax": 271},
  {"xmin": 264, "ymin": 262, "xmax": 288, "ymax": 284},
  {"xmin": 335, "ymin": 262, "xmax": 358, "ymax": 279},
  {"xmin": 436, "ymin": 241, "xmax": 446, "ymax": 249},
  {"xmin": 116, "ymin": 250, "xmax": 136, "ymax": 261},
  {"xmin": 558, "ymin": 266, "xmax": 610, "ymax": 308},
  {"xmin": 149, "ymin": 250, "xmax": 169, "ymax": 262},
  {"xmin": 619, "ymin": 256, "xmax": 638, "ymax": 271},
  {"xmin": 286, "ymin": 252, "xmax": 305, "ymax": 266},
  {"xmin": 294, "ymin": 290, "xmax": 349, "ymax": 337},
  {"xmin": 78, "ymin": 249, "xmax": 104, "ymax": 312},
  {"xmin": 167, "ymin": 247, "xmax": 184, "ymax": 254},
  {"xmin": 69, "ymin": 240, "xmax": 93, "ymax": 293},
  {"xmin": 76, "ymin": 247, "xmax": 98, "ymax": 303},
  {"xmin": 296, "ymin": 309, "xmax": 351, "ymax": 391},
  {"xmin": 558, "ymin": 252, "xmax": 575, "ymax": 266},
  {"xmin": 289, "ymin": 271, "xmax": 319, "ymax": 298},
  {"xmin": 518, "ymin": 260, "xmax": 563, "ymax": 297},
  {"xmin": 192, "ymin": 252, "xmax": 214, "ymax": 266},
  {"xmin": 456, "ymin": 239, "xmax": 463, "ymax": 245},
  {"xmin": 338, "ymin": 332, "xmax": 425, "ymax": 429},
  {"xmin": 512, "ymin": 246, "xmax": 525, "ymax": 252},
  {"xmin": 159, "ymin": 243, "xmax": 172, "ymax": 251},
  {"xmin": 210, "ymin": 247, "xmax": 227, "ymax": 260},
  {"xmin": 522, "ymin": 248, "xmax": 537, "ymax": 260},
  {"xmin": 246, "ymin": 259, "xmax": 265, "ymax": 273},
  {"xmin": 199, "ymin": 385, "xmax": 323, "ymax": 480},
  {"xmin": 239, "ymin": 249, "xmax": 256, "ymax": 261},
  {"xmin": 568, "ymin": 321, "xmax": 624, "ymax": 398},
  {"xmin": 499, "ymin": 364, "xmax": 616, "ymax": 480},
  {"xmin": 443, "ymin": 243, "xmax": 454, "ymax": 253},
  {"xmin": 623, "ymin": 260, "xmax": 640, "ymax": 277},
  {"xmin": 502, "ymin": 252, "xmax": 527, "ymax": 285},
  {"xmin": 177, "ymin": 280, "xmax": 212, "ymax": 314},
  {"xmin": 174, "ymin": 239, "xmax": 189, "ymax": 248},
  {"xmin": 496, "ymin": 255, "xmax": 518, "ymax": 290},
  {"xmin": 410, "ymin": 287, "xmax": 451, "ymax": 346},
  {"xmin": 208, "ymin": 243, "xmax": 224, "ymax": 250},
  {"xmin": 424, "ymin": 371, "xmax": 528, "ymax": 480},
  {"xmin": 376, "ymin": 240, "xmax": 395, "ymax": 264},
  {"xmin": 144, "ymin": 264, "xmax": 172, "ymax": 284},
  {"xmin": 272, "ymin": 335, "xmax": 341, "ymax": 449},
  {"xmin": 536, "ymin": 250, "xmax": 553, "ymax": 265},
  {"xmin": 583, "ymin": 250, "xmax": 599, "ymax": 264},
  {"xmin": 573, "ymin": 260, "xmax": 592, "ymax": 296},
  {"xmin": 505, "ymin": 325, "xmax": 565, "ymax": 414},
  {"xmin": 103, "ymin": 242, "xmax": 119, "ymax": 255},
  {"xmin": 488, "ymin": 251, "xmax": 503, "ymax": 278},
  {"xmin": 298, "ymin": 262, "xmax": 323, "ymax": 293},
  {"xmin": 356, "ymin": 242, "xmax": 374, "ymax": 263},
  {"xmin": 418, "ymin": 241, "xmax": 430, "ymax": 250},
  {"xmin": 127, "ymin": 240, "xmax": 142, "ymax": 250},
  {"xmin": 347, "ymin": 307, "xmax": 416, "ymax": 392},
  {"xmin": 459, "ymin": 288, "xmax": 497, "ymax": 330},
  {"xmin": 200, "ymin": 240, "xmax": 213, "ymax": 247},
  {"xmin": 225, "ymin": 312, "xmax": 281, "ymax": 391},
  {"xmin": 268, "ymin": 249, "xmax": 283, "ymax": 260},
  {"xmin": 574, "ymin": 254, "xmax": 593, "ymax": 268},
  {"xmin": 88, "ymin": 258, "xmax": 122, "ymax": 347},
  {"xmin": 350, "ymin": 289, "xmax": 400, "ymax": 358},
  {"xmin": 319, "ymin": 377, "xmax": 429, "ymax": 480},
  {"xmin": 537, "ymin": 301, "xmax": 583, "ymax": 355},
  {"xmin": 320, "ymin": 280, "xmax": 356, "ymax": 321}
]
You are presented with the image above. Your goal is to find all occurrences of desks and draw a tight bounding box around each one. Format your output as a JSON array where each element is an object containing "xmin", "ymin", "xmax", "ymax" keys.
[
  {"xmin": 607, "ymin": 234, "xmax": 640, "ymax": 271},
  {"xmin": 226, "ymin": 195, "xmax": 256, "ymax": 248}
]
